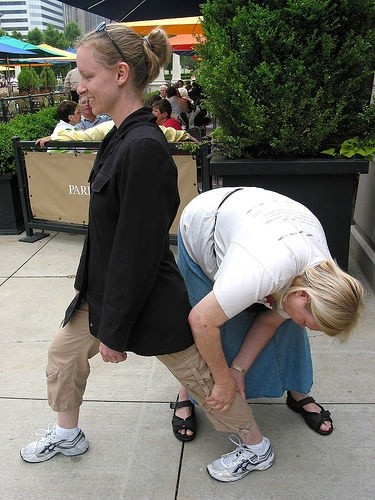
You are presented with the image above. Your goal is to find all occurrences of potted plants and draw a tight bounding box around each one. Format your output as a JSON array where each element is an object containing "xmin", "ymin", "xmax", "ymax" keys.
[
  {"xmin": 188, "ymin": 0, "xmax": 375, "ymax": 273},
  {"xmin": 17, "ymin": 66, "xmax": 58, "ymax": 95}
]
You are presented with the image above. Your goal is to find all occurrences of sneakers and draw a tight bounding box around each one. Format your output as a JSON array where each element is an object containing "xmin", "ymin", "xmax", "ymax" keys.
[
  {"xmin": 206, "ymin": 437, "xmax": 274, "ymax": 482},
  {"xmin": 20, "ymin": 424, "xmax": 89, "ymax": 463}
]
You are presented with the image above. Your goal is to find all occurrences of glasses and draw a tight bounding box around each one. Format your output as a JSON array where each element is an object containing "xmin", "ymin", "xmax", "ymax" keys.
[{"xmin": 95, "ymin": 21, "xmax": 128, "ymax": 62}]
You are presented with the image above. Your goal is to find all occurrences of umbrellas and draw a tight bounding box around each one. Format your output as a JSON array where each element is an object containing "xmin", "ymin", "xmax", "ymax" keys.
[{"xmin": 0, "ymin": 35, "xmax": 78, "ymax": 85}]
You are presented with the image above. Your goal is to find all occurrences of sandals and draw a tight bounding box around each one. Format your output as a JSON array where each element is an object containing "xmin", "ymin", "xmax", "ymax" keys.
[
  {"xmin": 170, "ymin": 393, "xmax": 196, "ymax": 442},
  {"xmin": 286, "ymin": 390, "xmax": 333, "ymax": 436}
]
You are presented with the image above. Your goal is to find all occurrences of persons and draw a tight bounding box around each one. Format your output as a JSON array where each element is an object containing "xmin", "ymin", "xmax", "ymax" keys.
[
  {"xmin": 18, "ymin": 22, "xmax": 274, "ymax": 483},
  {"xmin": 0, "ymin": 62, "xmax": 205, "ymax": 154},
  {"xmin": 170, "ymin": 186, "xmax": 365, "ymax": 442}
]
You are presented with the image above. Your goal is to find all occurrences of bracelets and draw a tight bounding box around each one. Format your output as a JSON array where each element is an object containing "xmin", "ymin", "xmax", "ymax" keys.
[{"xmin": 230, "ymin": 364, "xmax": 246, "ymax": 374}]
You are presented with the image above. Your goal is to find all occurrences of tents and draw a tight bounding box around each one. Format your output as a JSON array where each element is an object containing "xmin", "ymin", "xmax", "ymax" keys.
[{"xmin": 117, "ymin": 15, "xmax": 208, "ymax": 56}]
